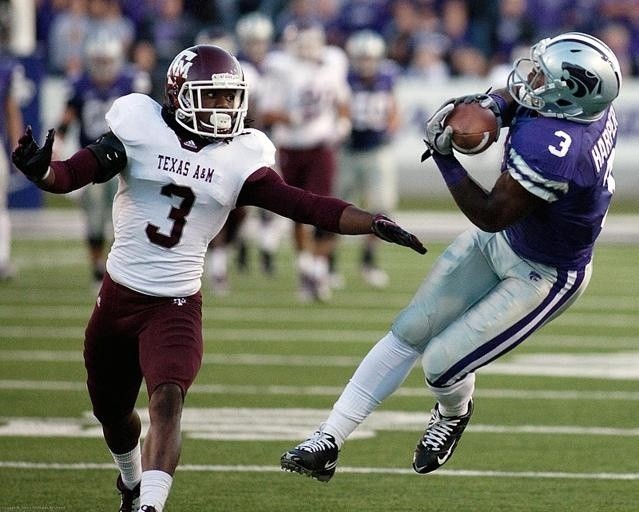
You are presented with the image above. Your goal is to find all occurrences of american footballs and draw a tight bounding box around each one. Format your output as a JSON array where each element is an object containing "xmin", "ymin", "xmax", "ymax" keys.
[{"xmin": 444, "ymin": 101, "xmax": 498, "ymax": 155}]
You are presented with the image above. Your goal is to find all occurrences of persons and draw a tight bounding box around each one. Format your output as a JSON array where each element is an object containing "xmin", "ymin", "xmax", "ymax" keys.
[
  {"xmin": 12, "ymin": 43, "xmax": 427, "ymax": 512},
  {"xmin": 278, "ymin": 31, "xmax": 623, "ymax": 482},
  {"xmin": 2, "ymin": 47, "xmax": 31, "ymax": 283},
  {"xmin": 41, "ymin": 0, "xmax": 639, "ymax": 81},
  {"xmin": 65, "ymin": 16, "xmax": 405, "ymax": 304}
]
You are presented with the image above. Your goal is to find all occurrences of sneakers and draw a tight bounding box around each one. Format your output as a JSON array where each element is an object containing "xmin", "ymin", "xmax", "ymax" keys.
[
  {"xmin": 116, "ymin": 472, "xmax": 156, "ymax": 512},
  {"xmin": 280, "ymin": 430, "xmax": 338, "ymax": 482},
  {"xmin": 209, "ymin": 233, "xmax": 389, "ymax": 301},
  {"xmin": 412, "ymin": 396, "xmax": 473, "ymax": 475}
]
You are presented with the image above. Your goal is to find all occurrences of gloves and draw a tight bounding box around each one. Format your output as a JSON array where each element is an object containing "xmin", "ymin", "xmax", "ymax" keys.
[
  {"xmin": 370, "ymin": 214, "xmax": 427, "ymax": 255},
  {"xmin": 423, "ymin": 94, "xmax": 501, "ymax": 155},
  {"xmin": 11, "ymin": 125, "xmax": 56, "ymax": 183}
]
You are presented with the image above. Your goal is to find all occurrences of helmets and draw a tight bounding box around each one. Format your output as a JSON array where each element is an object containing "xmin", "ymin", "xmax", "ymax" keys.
[
  {"xmin": 165, "ymin": 44, "xmax": 248, "ymax": 111},
  {"xmin": 527, "ymin": 31, "xmax": 622, "ymax": 119}
]
